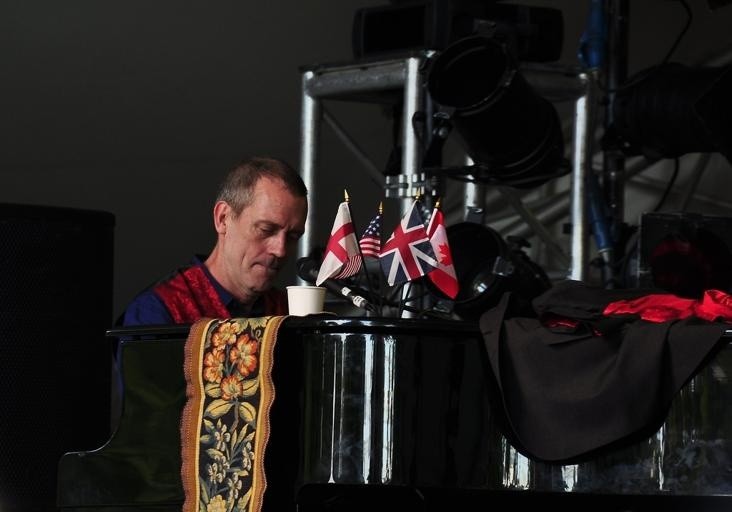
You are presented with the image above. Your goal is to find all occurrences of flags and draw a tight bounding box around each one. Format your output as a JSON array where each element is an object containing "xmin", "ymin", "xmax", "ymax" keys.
[{"xmin": 316, "ymin": 199, "xmax": 460, "ymax": 299}]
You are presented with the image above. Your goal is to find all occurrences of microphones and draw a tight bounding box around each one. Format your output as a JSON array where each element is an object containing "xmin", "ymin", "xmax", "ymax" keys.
[{"xmin": 295, "ymin": 256, "xmax": 377, "ymax": 313}]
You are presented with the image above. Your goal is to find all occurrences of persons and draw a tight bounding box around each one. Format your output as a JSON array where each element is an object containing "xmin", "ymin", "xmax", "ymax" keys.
[{"xmin": 110, "ymin": 157, "xmax": 309, "ymax": 435}]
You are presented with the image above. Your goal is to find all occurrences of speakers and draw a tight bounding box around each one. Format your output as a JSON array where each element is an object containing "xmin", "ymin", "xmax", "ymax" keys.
[{"xmin": 637, "ymin": 211, "xmax": 731, "ymax": 291}]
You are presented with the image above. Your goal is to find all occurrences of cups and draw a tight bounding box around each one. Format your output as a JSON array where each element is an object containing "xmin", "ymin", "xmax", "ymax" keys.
[{"xmin": 285, "ymin": 286, "xmax": 327, "ymax": 316}]
